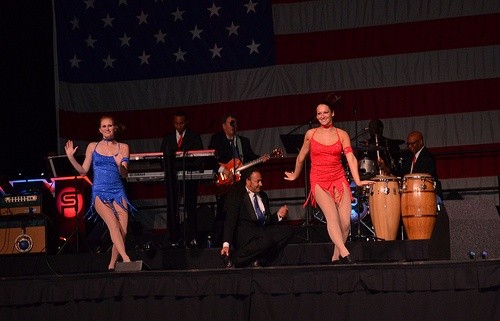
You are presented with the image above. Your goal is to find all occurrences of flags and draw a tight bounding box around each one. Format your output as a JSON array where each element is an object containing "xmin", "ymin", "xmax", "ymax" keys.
[{"xmin": 52, "ymin": 0, "xmax": 500, "ymax": 229}]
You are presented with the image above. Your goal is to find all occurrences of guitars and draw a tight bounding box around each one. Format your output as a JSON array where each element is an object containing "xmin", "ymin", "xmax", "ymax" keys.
[{"xmin": 205, "ymin": 146, "xmax": 284, "ymax": 196}]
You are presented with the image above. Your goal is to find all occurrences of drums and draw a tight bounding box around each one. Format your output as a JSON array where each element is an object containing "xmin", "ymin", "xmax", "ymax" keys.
[
  {"xmin": 366, "ymin": 175, "xmax": 402, "ymax": 242},
  {"xmin": 357, "ymin": 158, "xmax": 375, "ymax": 181},
  {"xmin": 399, "ymin": 172, "xmax": 438, "ymax": 241},
  {"xmin": 348, "ymin": 180, "xmax": 369, "ymax": 225}
]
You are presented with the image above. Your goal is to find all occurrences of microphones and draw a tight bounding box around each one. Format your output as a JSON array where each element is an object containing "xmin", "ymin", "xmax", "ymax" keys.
[{"xmin": 229, "ymin": 121, "xmax": 234, "ymax": 127}]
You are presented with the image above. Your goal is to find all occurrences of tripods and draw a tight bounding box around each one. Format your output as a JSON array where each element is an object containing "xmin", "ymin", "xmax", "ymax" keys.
[
  {"xmin": 51, "ymin": 170, "xmax": 97, "ymax": 272},
  {"xmin": 281, "ymin": 106, "xmax": 328, "ymax": 247},
  {"xmin": 349, "ymin": 111, "xmax": 375, "ymax": 237}
]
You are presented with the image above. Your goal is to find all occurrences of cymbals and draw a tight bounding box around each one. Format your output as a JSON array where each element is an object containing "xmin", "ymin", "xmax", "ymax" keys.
[
  {"xmin": 375, "ymin": 139, "xmax": 406, "ymax": 147},
  {"xmin": 351, "ymin": 143, "xmax": 387, "ymax": 152}
]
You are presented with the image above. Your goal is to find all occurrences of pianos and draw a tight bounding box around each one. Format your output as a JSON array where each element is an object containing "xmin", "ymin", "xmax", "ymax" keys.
[{"xmin": 126, "ymin": 150, "xmax": 220, "ymax": 244}]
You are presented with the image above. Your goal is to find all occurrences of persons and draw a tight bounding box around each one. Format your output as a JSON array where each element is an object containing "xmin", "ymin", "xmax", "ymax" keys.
[
  {"xmin": 65, "ymin": 116, "xmax": 139, "ymax": 272},
  {"xmin": 206, "ymin": 113, "xmax": 268, "ymax": 243},
  {"xmin": 284, "ymin": 101, "xmax": 377, "ymax": 265},
  {"xmin": 161, "ymin": 111, "xmax": 205, "ymax": 250},
  {"xmin": 390, "ymin": 131, "xmax": 436, "ymax": 178},
  {"xmin": 220, "ymin": 166, "xmax": 296, "ymax": 268},
  {"xmin": 367, "ymin": 119, "xmax": 400, "ymax": 162}
]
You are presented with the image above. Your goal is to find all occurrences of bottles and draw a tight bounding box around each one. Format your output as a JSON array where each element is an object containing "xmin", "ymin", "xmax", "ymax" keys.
[{"xmin": 207, "ymin": 236, "xmax": 213, "ymax": 248}]
